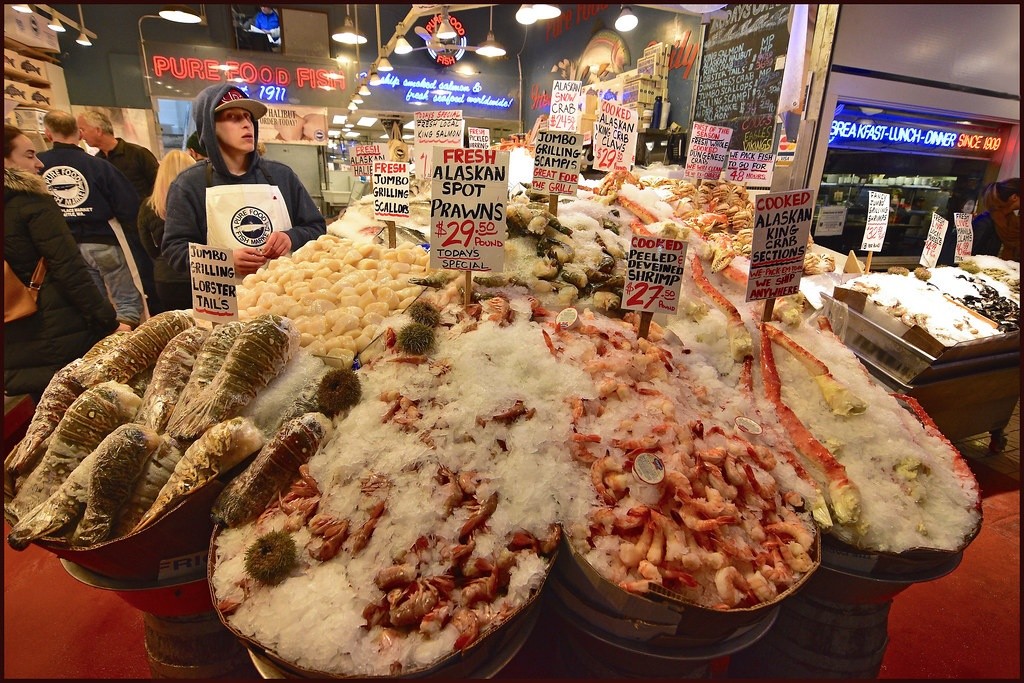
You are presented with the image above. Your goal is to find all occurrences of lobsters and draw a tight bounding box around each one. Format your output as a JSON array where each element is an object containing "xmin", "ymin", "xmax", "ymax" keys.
[
  {"xmin": 3, "ymin": 308, "xmax": 335, "ymax": 552},
  {"xmin": 275, "ymin": 355, "xmax": 565, "ymax": 653}
]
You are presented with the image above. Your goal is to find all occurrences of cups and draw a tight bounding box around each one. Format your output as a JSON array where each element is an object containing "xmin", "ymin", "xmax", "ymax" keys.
[{"xmin": 643, "ymin": 110, "xmax": 653, "ymax": 128}]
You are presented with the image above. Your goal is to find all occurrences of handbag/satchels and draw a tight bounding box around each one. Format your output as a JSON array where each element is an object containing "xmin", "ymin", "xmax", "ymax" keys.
[{"xmin": 4, "ymin": 254, "xmax": 48, "ymax": 324}]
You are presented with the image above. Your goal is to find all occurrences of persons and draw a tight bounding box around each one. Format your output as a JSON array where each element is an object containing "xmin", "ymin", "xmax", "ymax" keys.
[
  {"xmin": 243, "ymin": 7, "xmax": 282, "ymax": 53},
  {"xmin": 944, "ymin": 178, "xmax": 1020, "ymax": 268},
  {"xmin": 78, "ymin": 112, "xmax": 159, "ymax": 312},
  {"xmin": 136, "ymin": 131, "xmax": 210, "ymax": 311},
  {"xmin": 4, "ymin": 125, "xmax": 132, "ymax": 401},
  {"xmin": 161, "ymin": 83, "xmax": 326, "ymax": 311},
  {"xmin": 32, "ymin": 110, "xmax": 145, "ymax": 329}
]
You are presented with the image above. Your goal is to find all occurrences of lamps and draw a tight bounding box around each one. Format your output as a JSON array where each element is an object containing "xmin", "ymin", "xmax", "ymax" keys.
[
  {"xmin": 345, "ymin": 113, "xmax": 354, "ymax": 128},
  {"xmin": 12, "ymin": 4, "xmax": 32, "ymax": 13},
  {"xmin": 476, "ymin": 6, "xmax": 506, "ymax": 57},
  {"xmin": 615, "ymin": 5, "xmax": 638, "ymax": 32},
  {"xmin": 358, "ymin": 77, "xmax": 371, "ymax": 95},
  {"xmin": 332, "ymin": 5, "xmax": 367, "ymax": 45},
  {"xmin": 515, "ymin": 4, "xmax": 561, "ymax": 25},
  {"xmin": 369, "ymin": 63, "xmax": 381, "ymax": 86},
  {"xmin": 347, "ymin": 99, "xmax": 359, "ymax": 111},
  {"xmin": 376, "ymin": 45, "xmax": 394, "ymax": 71},
  {"xmin": 352, "ymin": 86, "xmax": 364, "ymax": 103},
  {"xmin": 47, "ymin": 9, "xmax": 66, "ymax": 32},
  {"xmin": 76, "ymin": 4, "xmax": 92, "ymax": 46},
  {"xmin": 436, "ymin": 6, "xmax": 457, "ymax": 39},
  {"xmin": 394, "ymin": 22, "xmax": 413, "ymax": 54},
  {"xmin": 158, "ymin": 4, "xmax": 203, "ymax": 25}
]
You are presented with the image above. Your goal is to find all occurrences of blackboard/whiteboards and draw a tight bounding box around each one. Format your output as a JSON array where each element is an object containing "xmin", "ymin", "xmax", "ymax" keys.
[{"xmin": 692, "ymin": 4, "xmax": 795, "ymax": 154}]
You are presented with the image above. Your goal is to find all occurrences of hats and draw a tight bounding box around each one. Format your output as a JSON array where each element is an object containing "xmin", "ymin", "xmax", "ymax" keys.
[
  {"xmin": 186, "ymin": 130, "xmax": 207, "ymax": 157},
  {"xmin": 214, "ymin": 89, "xmax": 268, "ymax": 121}
]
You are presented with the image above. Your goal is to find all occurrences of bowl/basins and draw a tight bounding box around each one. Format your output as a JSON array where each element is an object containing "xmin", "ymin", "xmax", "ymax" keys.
[
  {"xmin": 734, "ymin": 416, "xmax": 763, "ymax": 441},
  {"xmin": 248, "ymin": 578, "xmax": 547, "ymax": 683},
  {"xmin": 556, "ymin": 308, "xmax": 580, "ymax": 330},
  {"xmin": 59, "ymin": 557, "xmax": 217, "ymax": 621},
  {"xmin": 632, "ymin": 453, "xmax": 665, "ymax": 485},
  {"xmin": 547, "ymin": 596, "xmax": 781, "ymax": 678},
  {"xmin": 806, "ymin": 551, "xmax": 964, "ymax": 605}
]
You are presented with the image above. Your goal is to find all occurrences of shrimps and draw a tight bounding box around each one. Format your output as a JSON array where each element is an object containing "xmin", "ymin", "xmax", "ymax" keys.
[
  {"xmin": 409, "ymin": 202, "xmax": 632, "ymax": 313},
  {"xmin": 543, "ymin": 323, "xmax": 815, "ymax": 611}
]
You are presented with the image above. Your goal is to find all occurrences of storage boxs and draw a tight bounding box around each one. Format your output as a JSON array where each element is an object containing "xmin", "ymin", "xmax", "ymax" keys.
[{"xmin": 832, "ymin": 257, "xmax": 1019, "ymax": 364}]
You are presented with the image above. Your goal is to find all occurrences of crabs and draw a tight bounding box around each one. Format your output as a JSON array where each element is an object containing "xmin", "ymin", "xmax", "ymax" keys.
[{"xmin": 577, "ymin": 168, "xmax": 984, "ymax": 529}]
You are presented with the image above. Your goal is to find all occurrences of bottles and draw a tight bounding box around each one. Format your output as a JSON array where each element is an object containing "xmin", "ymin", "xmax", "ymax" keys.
[{"xmin": 653, "ymin": 96, "xmax": 671, "ymax": 130}]
